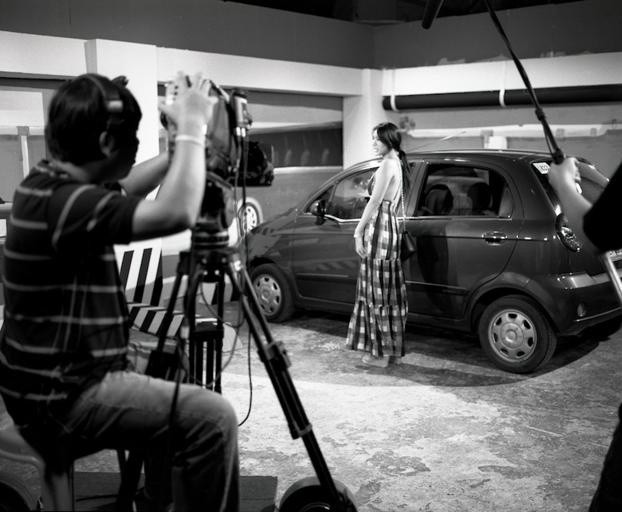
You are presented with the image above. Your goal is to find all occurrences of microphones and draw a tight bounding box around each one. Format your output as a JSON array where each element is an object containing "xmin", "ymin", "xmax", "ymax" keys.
[{"xmin": 421, "ymin": 1, "xmax": 444, "ymax": 30}]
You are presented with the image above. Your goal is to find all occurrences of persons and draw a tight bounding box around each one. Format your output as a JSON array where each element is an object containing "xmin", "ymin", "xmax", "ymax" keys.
[
  {"xmin": 0, "ymin": 71, "xmax": 242, "ymax": 511},
  {"xmin": 546, "ymin": 152, "xmax": 621, "ymax": 512},
  {"xmin": 343, "ymin": 122, "xmax": 413, "ymax": 368}
]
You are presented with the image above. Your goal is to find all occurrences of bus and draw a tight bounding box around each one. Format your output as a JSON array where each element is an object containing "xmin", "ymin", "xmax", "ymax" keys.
[{"xmin": 222, "ymin": 119, "xmax": 342, "ymax": 234}]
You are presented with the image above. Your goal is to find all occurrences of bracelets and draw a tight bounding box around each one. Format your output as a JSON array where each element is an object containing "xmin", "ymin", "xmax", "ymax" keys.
[
  {"xmin": 352, "ymin": 231, "xmax": 364, "ymax": 239},
  {"xmin": 173, "ymin": 134, "xmax": 206, "ymax": 147}
]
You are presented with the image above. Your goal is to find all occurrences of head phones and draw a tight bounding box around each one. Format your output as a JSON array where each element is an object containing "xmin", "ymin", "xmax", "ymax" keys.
[{"xmin": 71, "ymin": 72, "xmax": 124, "ymax": 152}]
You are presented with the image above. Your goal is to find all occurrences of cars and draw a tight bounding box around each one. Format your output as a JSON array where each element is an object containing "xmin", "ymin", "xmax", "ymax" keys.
[{"xmin": 229, "ymin": 145, "xmax": 622, "ymax": 375}]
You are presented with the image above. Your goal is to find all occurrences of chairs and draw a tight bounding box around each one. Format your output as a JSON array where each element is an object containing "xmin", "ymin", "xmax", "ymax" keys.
[
  {"xmin": 467, "ymin": 180, "xmax": 493, "ymax": 214},
  {"xmin": 425, "ymin": 182, "xmax": 454, "ymax": 214}
]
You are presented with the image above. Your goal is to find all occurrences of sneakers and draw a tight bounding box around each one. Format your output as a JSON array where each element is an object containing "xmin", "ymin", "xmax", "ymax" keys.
[{"xmin": 362, "ymin": 355, "xmax": 403, "ymax": 368}]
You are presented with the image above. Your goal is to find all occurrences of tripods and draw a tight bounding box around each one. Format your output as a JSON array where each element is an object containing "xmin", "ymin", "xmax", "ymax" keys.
[{"xmin": 111, "ymin": 180, "xmax": 351, "ymax": 512}]
